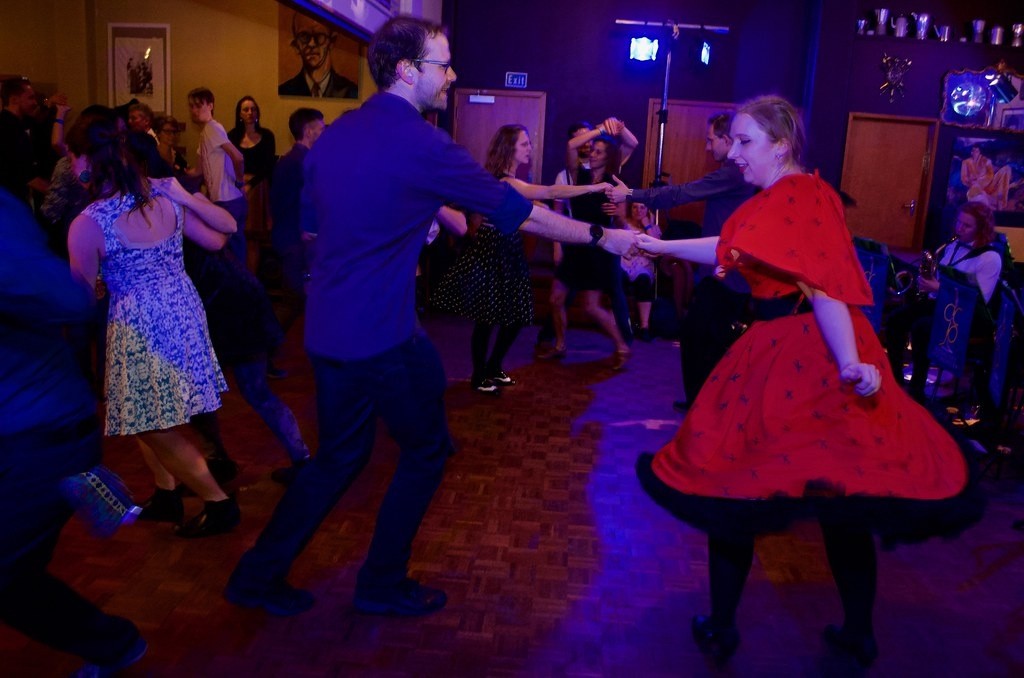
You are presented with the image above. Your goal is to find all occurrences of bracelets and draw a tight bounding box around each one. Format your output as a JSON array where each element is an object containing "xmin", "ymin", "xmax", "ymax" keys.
[{"xmin": 643, "ymin": 224, "xmax": 652, "ymax": 230}]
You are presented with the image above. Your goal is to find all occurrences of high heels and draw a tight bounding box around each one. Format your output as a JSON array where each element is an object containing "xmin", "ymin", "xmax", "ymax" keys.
[
  {"xmin": 693, "ymin": 614, "xmax": 740, "ymax": 669},
  {"xmin": 825, "ymin": 623, "xmax": 879, "ymax": 669}
]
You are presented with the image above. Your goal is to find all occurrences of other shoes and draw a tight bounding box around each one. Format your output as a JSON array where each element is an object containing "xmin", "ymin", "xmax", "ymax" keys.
[
  {"xmin": 673, "ymin": 400, "xmax": 687, "ymax": 413},
  {"xmin": 350, "ymin": 578, "xmax": 447, "ymax": 619},
  {"xmin": 178, "ymin": 452, "xmax": 236, "ymax": 496},
  {"xmin": 612, "ymin": 349, "xmax": 632, "ymax": 370},
  {"xmin": 271, "ymin": 459, "xmax": 312, "ymax": 483},
  {"xmin": 630, "ymin": 323, "xmax": 651, "ymax": 342},
  {"xmin": 174, "ymin": 493, "xmax": 241, "ymax": 538},
  {"xmin": 136, "ymin": 487, "xmax": 179, "ymax": 522},
  {"xmin": 536, "ymin": 347, "xmax": 568, "ymax": 361},
  {"xmin": 223, "ymin": 572, "xmax": 315, "ymax": 617},
  {"xmin": 69, "ymin": 637, "xmax": 148, "ymax": 678}
]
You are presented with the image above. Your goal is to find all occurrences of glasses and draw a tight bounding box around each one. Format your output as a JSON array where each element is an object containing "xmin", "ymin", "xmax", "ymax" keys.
[{"xmin": 405, "ymin": 60, "xmax": 451, "ymax": 73}]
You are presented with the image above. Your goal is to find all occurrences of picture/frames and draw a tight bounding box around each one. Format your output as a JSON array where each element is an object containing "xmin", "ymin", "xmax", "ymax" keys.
[{"xmin": 109, "ymin": 22, "xmax": 171, "ymax": 120}]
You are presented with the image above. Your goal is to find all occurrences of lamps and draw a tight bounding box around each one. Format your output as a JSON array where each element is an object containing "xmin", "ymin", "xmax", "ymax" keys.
[
  {"xmin": 688, "ymin": 22, "xmax": 713, "ymax": 69},
  {"xmin": 630, "ymin": 19, "xmax": 658, "ymax": 61}
]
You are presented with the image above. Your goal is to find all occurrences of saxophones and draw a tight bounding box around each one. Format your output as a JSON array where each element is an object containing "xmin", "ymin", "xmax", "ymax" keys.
[{"xmin": 890, "ymin": 233, "xmax": 958, "ymax": 306}]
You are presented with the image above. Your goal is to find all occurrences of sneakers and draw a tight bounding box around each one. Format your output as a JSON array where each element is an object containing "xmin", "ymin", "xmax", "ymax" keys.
[
  {"xmin": 471, "ymin": 379, "xmax": 499, "ymax": 394},
  {"xmin": 488, "ymin": 370, "xmax": 512, "ymax": 385}
]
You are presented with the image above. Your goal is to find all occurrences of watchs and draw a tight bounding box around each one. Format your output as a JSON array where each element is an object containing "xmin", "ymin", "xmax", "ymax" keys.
[
  {"xmin": 589, "ymin": 224, "xmax": 604, "ymax": 245},
  {"xmin": 626, "ymin": 188, "xmax": 633, "ymax": 201}
]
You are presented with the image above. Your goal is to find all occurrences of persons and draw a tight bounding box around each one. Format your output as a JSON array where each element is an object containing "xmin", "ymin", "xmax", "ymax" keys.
[
  {"xmin": 222, "ymin": 14, "xmax": 642, "ymax": 622},
  {"xmin": 431, "ymin": 123, "xmax": 663, "ymax": 395},
  {"xmin": 630, "ymin": 94, "xmax": 988, "ymax": 670},
  {"xmin": 0, "ymin": 72, "xmax": 332, "ymax": 678},
  {"xmin": 960, "ymin": 145, "xmax": 1024, "ymax": 210},
  {"xmin": 604, "ymin": 110, "xmax": 759, "ymax": 409},
  {"xmin": 883, "ymin": 201, "xmax": 1002, "ymax": 405}
]
